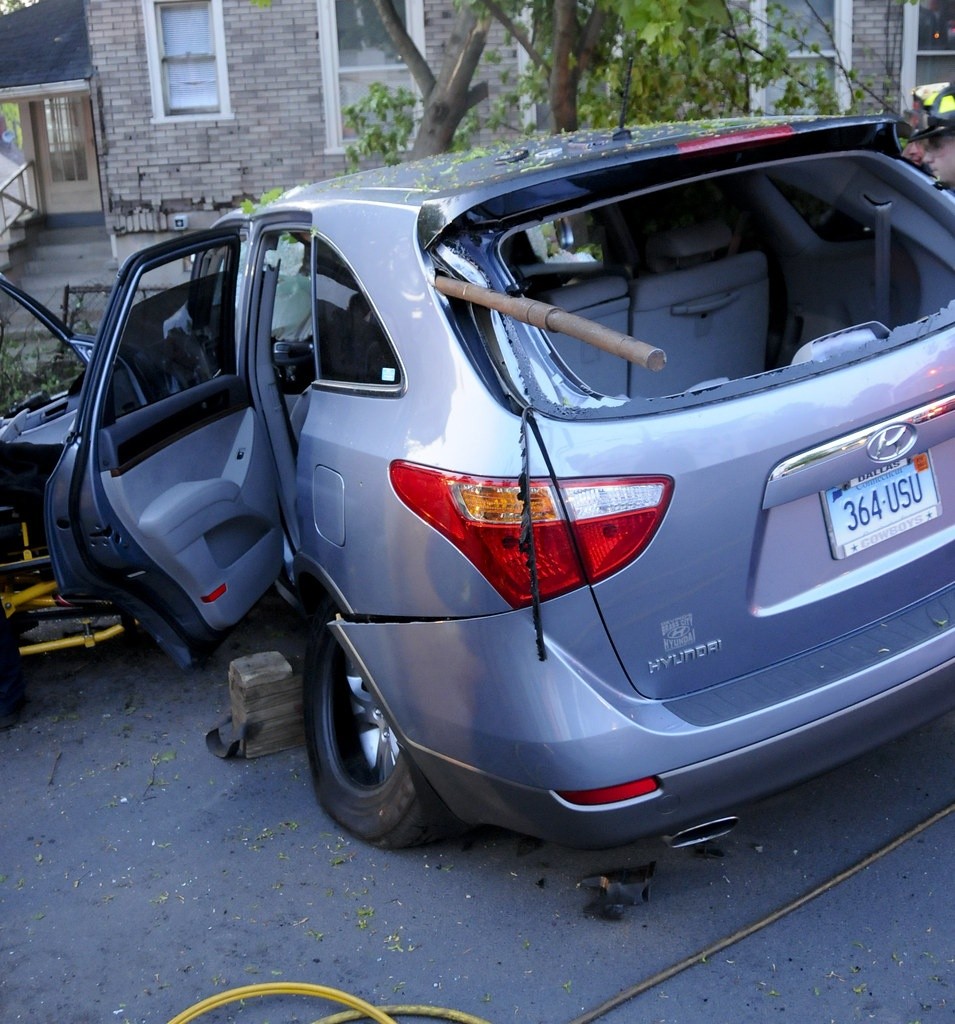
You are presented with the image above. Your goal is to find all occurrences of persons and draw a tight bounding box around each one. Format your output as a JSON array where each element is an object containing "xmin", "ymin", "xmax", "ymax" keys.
[
  {"xmin": 907, "ymin": 86, "xmax": 955, "ymax": 193},
  {"xmin": 901, "ymin": 138, "xmax": 933, "ymax": 177}
]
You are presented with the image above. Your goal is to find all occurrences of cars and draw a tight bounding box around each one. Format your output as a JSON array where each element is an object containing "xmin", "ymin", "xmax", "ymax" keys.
[{"xmin": 0, "ymin": 114, "xmax": 955, "ymax": 857}]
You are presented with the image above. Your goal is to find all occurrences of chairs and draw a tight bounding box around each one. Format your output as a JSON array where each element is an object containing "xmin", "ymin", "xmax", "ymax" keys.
[
  {"xmin": 546, "ymin": 253, "xmax": 629, "ymax": 406},
  {"xmin": 632, "ymin": 250, "xmax": 772, "ymax": 396}
]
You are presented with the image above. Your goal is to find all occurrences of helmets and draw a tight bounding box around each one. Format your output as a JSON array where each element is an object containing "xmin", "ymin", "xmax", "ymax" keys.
[
  {"xmin": 907, "ymin": 87, "xmax": 955, "ymax": 143},
  {"xmin": 897, "ymin": 109, "xmax": 930, "ymax": 138}
]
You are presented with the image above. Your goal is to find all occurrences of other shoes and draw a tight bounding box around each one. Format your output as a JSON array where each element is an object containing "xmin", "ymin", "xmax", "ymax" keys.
[{"xmin": 0, "ymin": 692, "xmax": 26, "ymax": 728}]
